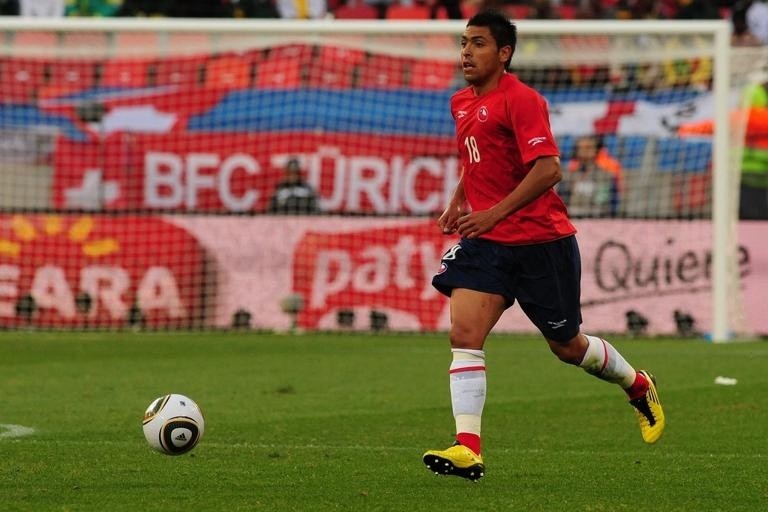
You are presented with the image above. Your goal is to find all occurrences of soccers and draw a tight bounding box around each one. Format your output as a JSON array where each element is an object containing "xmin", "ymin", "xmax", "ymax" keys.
[{"xmin": 143, "ymin": 394, "xmax": 204, "ymax": 455}]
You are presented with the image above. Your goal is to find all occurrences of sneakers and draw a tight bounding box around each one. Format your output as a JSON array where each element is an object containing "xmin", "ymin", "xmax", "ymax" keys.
[
  {"xmin": 422, "ymin": 440, "xmax": 484, "ymax": 482},
  {"xmin": 629, "ymin": 369, "xmax": 664, "ymax": 444}
]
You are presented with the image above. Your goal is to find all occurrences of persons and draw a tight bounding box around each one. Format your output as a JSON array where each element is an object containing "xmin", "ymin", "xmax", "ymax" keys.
[
  {"xmin": 553, "ymin": 133, "xmax": 617, "ymax": 220},
  {"xmin": 419, "ymin": 8, "xmax": 670, "ymax": 483},
  {"xmin": 265, "ymin": 158, "xmax": 318, "ymax": 217},
  {"xmin": 1, "ymin": 2, "xmax": 766, "ymax": 116}
]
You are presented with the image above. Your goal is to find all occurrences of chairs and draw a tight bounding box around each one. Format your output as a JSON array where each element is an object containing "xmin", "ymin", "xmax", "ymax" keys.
[{"xmin": 0, "ymin": 7, "xmax": 480, "ymax": 97}]
